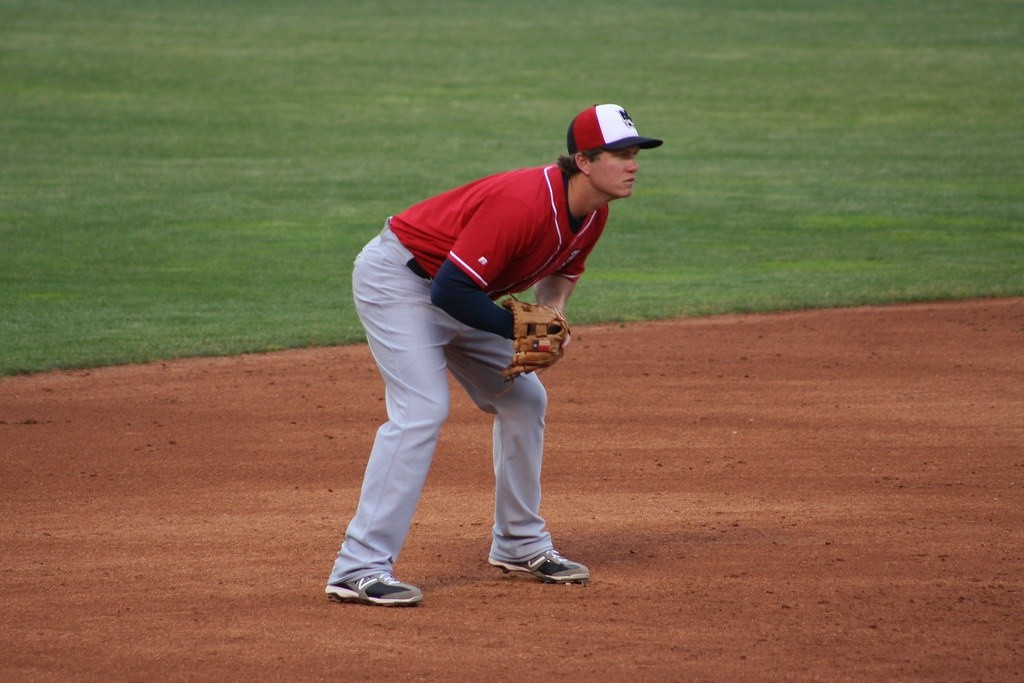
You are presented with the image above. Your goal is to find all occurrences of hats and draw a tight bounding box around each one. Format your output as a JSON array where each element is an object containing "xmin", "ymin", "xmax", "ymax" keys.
[{"xmin": 567, "ymin": 103, "xmax": 663, "ymax": 155}]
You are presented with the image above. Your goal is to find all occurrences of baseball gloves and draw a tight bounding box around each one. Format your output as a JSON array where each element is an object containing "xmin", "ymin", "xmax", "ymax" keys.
[{"xmin": 499, "ymin": 298, "xmax": 571, "ymax": 382}]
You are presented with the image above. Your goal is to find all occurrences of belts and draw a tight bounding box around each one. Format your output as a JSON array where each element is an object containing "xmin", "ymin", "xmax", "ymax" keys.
[{"xmin": 407, "ymin": 259, "xmax": 430, "ymax": 281}]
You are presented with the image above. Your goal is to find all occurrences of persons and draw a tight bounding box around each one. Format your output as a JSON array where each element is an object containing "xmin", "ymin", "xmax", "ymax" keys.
[{"xmin": 324, "ymin": 104, "xmax": 663, "ymax": 607}]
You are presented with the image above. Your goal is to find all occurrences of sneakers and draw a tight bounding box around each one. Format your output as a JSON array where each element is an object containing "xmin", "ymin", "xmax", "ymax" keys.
[
  {"xmin": 488, "ymin": 548, "xmax": 590, "ymax": 586},
  {"xmin": 324, "ymin": 571, "xmax": 424, "ymax": 607}
]
